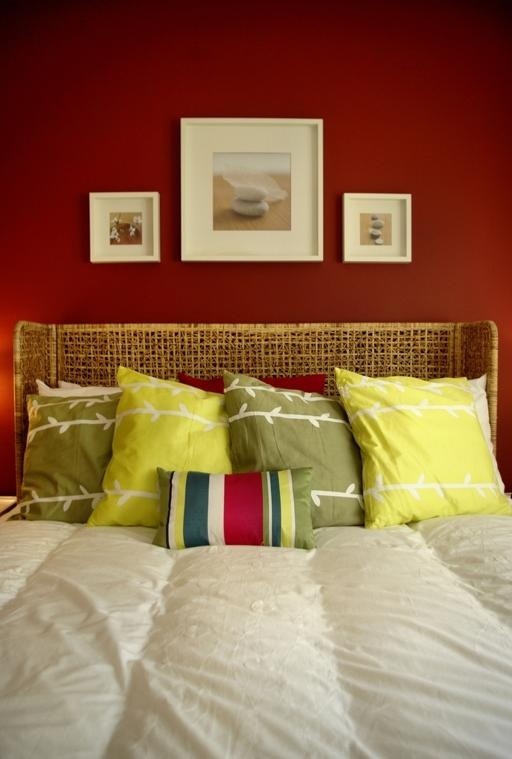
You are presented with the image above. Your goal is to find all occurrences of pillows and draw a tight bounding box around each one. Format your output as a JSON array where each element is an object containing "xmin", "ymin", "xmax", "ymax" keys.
[
  {"xmin": 468, "ymin": 370, "xmax": 505, "ymax": 494},
  {"xmin": 16, "ymin": 389, "xmax": 121, "ymax": 526},
  {"xmin": 57, "ymin": 378, "xmax": 83, "ymax": 389},
  {"xmin": 83, "ymin": 365, "xmax": 233, "ymax": 528},
  {"xmin": 174, "ymin": 369, "xmax": 327, "ymax": 397},
  {"xmin": 155, "ymin": 466, "xmax": 317, "ymax": 550},
  {"xmin": 34, "ymin": 378, "xmax": 123, "ymax": 400},
  {"xmin": 222, "ymin": 369, "xmax": 367, "ymax": 529},
  {"xmin": 333, "ymin": 366, "xmax": 511, "ymax": 531}
]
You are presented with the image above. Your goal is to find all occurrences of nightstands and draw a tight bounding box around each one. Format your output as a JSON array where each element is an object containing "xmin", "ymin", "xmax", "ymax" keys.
[{"xmin": 1, "ymin": 492, "xmax": 19, "ymax": 519}]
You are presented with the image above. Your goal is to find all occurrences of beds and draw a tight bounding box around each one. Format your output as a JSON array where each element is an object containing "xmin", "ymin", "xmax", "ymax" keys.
[{"xmin": 0, "ymin": 316, "xmax": 511, "ymax": 757}]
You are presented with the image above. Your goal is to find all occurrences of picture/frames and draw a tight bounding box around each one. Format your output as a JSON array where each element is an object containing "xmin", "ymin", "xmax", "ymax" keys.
[
  {"xmin": 338, "ymin": 189, "xmax": 416, "ymax": 265},
  {"xmin": 177, "ymin": 115, "xmax": 326, "ymax": 263},
  {"xmin": 88, "ymin": 190, "xmax": 162, "ymax": 265}
]
